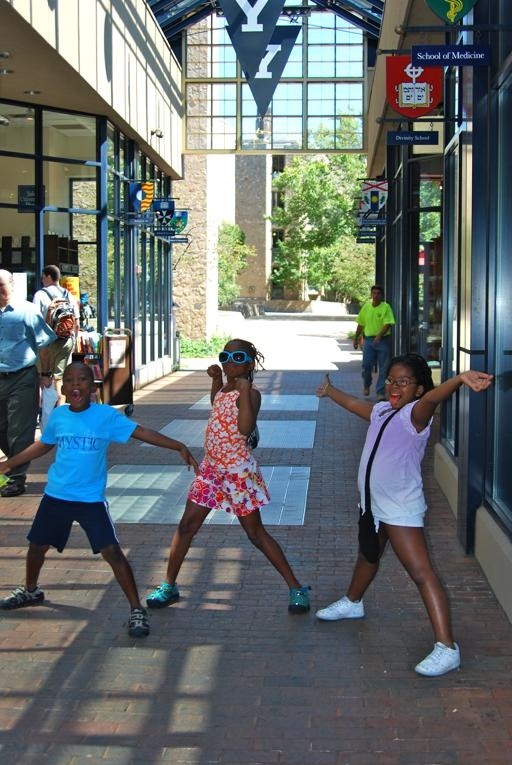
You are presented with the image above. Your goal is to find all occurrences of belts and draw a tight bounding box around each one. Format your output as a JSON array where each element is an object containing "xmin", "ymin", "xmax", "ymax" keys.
[{"xmin": 365, "ymin": 336, "xmax": 391, "ymax": 339}]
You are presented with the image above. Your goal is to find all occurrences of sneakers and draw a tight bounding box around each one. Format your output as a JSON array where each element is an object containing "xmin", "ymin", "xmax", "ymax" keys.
[
  {"xmin": 128, "ymin": 607, "xmax": 150, "ymax": 636},
  {"xmin": 377, "ymin": 393, "xmax": 386, "ymax": 401},
  {"xmin": 289, "ymin": 587, "xmax": 309, "ymax": 613},
  {"xmin": 364, "ymin": 387, "xmax": 369, "ymax": 396},
  {"xmin": 414, "ymin": 642, "xmax": 460, "ymax": 676},
  {"xmin": 146, "ymin": 582, "xmax": 179, "ymax": 606},
  {"xmin": 1, "ymin": 480, "xmax": 25, "ymax": 496},
  {"xmin": 0, "ymin": 586, "xmax": 44, "ymax": 609},
  {"xmin": 315, "ymin": 596, "xmax": 365, "ymax": 621}
]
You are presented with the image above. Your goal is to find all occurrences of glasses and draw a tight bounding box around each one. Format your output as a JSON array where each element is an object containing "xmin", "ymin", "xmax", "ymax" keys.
[
  {"xmin": 385, "ymin": 377, "xmax": 415, "ymax": 386},
  {"xmin": 219, "ymin": 351, "xmax": 253, "ymax": 364}
]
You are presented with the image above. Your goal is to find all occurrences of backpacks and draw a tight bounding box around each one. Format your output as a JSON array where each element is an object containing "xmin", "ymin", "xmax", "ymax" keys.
[
  {"xmin": 40, "ymin": 287, "xmax": 75, "ymax": 340},
  {"xmin": 247, "ymin": 425, "xmax": 259, "ymax": 450}
]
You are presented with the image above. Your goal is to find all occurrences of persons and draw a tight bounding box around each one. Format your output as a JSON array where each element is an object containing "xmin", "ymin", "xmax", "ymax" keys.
[
  {"xmin": 0, "ymin": 362, "xmax": 200, "ymax": 638},
  {"xmin": 314, "ymin": 353, "xmax": 494, "ymax": 679},
  {"xmin": 0, "ymin": 270, "xmax": 56, "ymax": 498},
  {"xmin": 146, "ymin": 338, "xmax": 313, "ymax": 615},
  {"xmin": 31, "ymin": 264, "xmax": 81, "ymax": 426},
  {"xmin": 351, "ymin": 284, "xmax": 396, "ymax": 402}
]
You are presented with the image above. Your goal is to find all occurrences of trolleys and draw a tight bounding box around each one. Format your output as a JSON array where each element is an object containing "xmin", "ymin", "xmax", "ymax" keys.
[{"xmin": 71, "ymin": 328, "xmax": 134, "ymax": 414}]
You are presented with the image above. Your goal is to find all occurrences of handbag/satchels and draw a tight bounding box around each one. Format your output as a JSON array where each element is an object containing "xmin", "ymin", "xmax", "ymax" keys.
[{"xmin": 358, "ymin": 513, "xmax": 379, "ymax": 565}]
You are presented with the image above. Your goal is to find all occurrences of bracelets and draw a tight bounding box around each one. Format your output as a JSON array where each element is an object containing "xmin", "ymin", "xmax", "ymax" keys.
[{"xmin": 39, "ymin": 370, "xmax": 53, "ymax": 378}]
